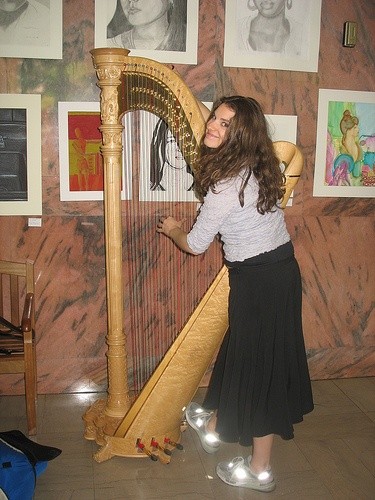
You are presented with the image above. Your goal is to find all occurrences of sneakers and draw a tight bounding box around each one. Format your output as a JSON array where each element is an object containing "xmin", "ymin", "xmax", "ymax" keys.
[
  {"xmin": 186, "ymin": 402, "xmax": 221, "ymax": 454},
  {"xmin": 216, "ymin": 456, "xmax": 276, "ymax": 492}
]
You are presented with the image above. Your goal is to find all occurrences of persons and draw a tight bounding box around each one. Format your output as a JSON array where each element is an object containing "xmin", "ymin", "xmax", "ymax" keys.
[{"xmin": 157, "ymin": 96, "xmax": 314, "ymax": 492}]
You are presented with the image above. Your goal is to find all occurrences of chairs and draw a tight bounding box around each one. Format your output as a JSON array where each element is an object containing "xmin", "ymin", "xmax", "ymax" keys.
[{"xmin": 0, "ymin": 257, "xmax": 36, "ymax": 436}]
